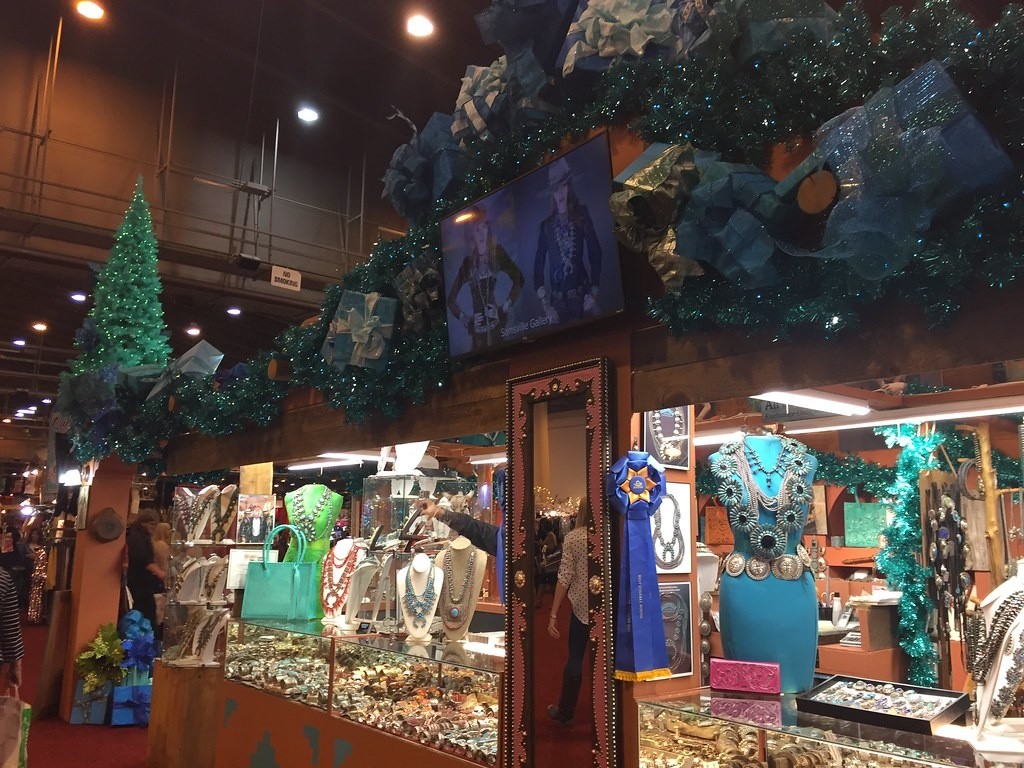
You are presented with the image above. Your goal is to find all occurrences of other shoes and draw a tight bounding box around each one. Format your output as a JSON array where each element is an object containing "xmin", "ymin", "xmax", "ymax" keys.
[{"xmin": 547, "ymin": 705, "xmax": 573, "ymax": 727}]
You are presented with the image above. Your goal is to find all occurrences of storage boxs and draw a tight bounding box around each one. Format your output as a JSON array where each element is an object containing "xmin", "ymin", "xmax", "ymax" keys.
[
  {"xmin": 700, "ymin": 515, "xmax": 705, "ymax": 544},
  {"xmin": 830, "ymin": 535, "xmax": 845, "ymax": 547},
  {"xmin": 873, "ymin": 589, "xmax": 904, "ymax": 600},
  {"xmin": 708, "ymin": 656, "xmax": 781, "ymax": 696},
  {"xmin": 818, "ymin": 619, "xmax": 861, "ymax": 647},
  {"xmin": 359, "ymin": 475, "xmax": 493, "ymax": 640},
  {"xmin": 706, "ymin": 505, "xmax": 735, "ymax": 545},
  {"xmin": 853, "ymin": 569, "xmax": 868, "ymax": 582},
  {"xmin": 111, "ymin": 685, "xmax": 152, "ymax": 727},
  {"xmin": 843, "ymin": 500, "xmax": 888, "ymax": 548},
  {"xmin": 119, "ymin": 664, "xmax": 150, "ymax": 686},
  {"xmin": 68, "ymin": 678, "xmax": 113, "ymax": 726},
  {"xmin": 840, "ymin": 632, "xmax": 862, "ymax": 647},
  {"xmin": 795, "ymin": 674, "xmax": 971, "ymax": 737},
  {"xmin": 710, "ymin": 690, "xmax": 783, "ymax": 727},
  {"xmin": 818, "ymin": 607, "xmax": 832, "ymax": 621}
]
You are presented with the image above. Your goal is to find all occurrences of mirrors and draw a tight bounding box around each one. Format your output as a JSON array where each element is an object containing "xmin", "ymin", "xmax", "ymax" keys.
[{"xmin": 503, "ymin": 354, "xmax": 622, "ymax": 768}]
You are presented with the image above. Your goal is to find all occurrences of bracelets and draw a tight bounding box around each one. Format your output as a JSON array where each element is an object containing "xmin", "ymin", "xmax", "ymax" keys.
[
  {"xmin": 536, "ymin": 296, "xmax": 550, "ymax": 304},
  {"xmin": 550, "ymin": 615, "xmax": 556, "ymax": 619}
]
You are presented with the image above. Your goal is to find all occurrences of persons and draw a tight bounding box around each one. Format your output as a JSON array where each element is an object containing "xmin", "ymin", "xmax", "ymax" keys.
[
  {"xmin": 545, "ymin": 495, "xmax": 592, "ymax": 727},
  {"xmin": 281, "ymin": 483, "xmax": 343, "ymax": 619},
  {"xmin": 0, "ymin": 565, "xmax": 25, "ymax": 697},
  {"xmin": 238, "ymin": 506, "xmax": 274, "ymax": 544},
  {"xmin": 151, "ymin": 522, "xmax": 172, "ymax": 625},
  {"xmin": 703, "ymin": 434, "xmax": 820, "ymax": 695},
  {"xmin": 127, "ymin": 507, "xmax": 167, "ymax": 628},
  {"xmin": 443, "ymin": 208, "xmax": 527, "ymax": 348},
  {"xmin": 21, "ymin": 528, "xmax": 49, "ymax": 613},
  {"xmin": 414, "ymin": 498, "xmax": 498, "ymax": 557},
  {"xmin": 532, "ymin": 158, "xmax": 604, "ymax": 323}
]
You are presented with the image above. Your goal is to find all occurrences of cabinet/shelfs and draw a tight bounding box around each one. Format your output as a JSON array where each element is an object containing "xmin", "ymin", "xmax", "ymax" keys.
[
  {"xmin": 141, "ymin": 485, "xmax": 276, "ymax": 768},
  {"xmin": 819, "ymin": 600, "xmax": 908, "ymax": 682}
]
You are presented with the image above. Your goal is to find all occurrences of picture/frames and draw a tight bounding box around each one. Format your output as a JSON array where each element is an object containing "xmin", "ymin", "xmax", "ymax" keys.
[
  {"xmin": 650, "ymin": 480, "xmax": 692, "ymax": 576},
  {"xmin": 802, "ymin": 483, "xmax": 829, "ymax": 536},
  {"xmin": 643, "ymin": 581, "xmax": 694, "ymax": 682},
  {"xmin": 640, "ymin": 405, "xmax": 691, "ymax": 471}
]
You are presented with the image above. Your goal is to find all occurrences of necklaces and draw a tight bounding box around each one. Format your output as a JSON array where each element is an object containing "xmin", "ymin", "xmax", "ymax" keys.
[
  {"xmin": 477, "ymin": 250, "xmax": 494, "ymax": 345},
  {"xmin": 292, "ymin": 485, "xmax": 334, "ymax": 541},
  {"xmin": 401, "ymin": 563, "xmax": 437, "ymax": 631},
  {"xmin": 320, "ymin": 544, "xmax": 359, "ymax": 619},
  {"xmin": 204, "ymin": 564, "xmax": 228, "ymax": 598},
  {"xmin": 178, "ymin": 608, "xmax": 202, "ymax": 651},
  {"xmin": 551, "ymin": 211, "xmax": 577, "ymax": 276},
  {"xmin": 174, "ymin": 561, "xmax": 196, "ymax": 591},
  {"xmin": 742, "ymin": 435, "xmax": 789, "ymax": 488},
  {"xmin": 177, "ymin": 490, "xmax": 215, "ymax": 536},
  {"xmin": 440, "ymin": 547, "xmax": 478, "ymax": 629},
  {"xmin": 196, "ymin": 610, "xmax": 230, "ymax": 654},
  {"xmin": 211, "ymin": 490, "xmax": 239, "ymax": 540}
]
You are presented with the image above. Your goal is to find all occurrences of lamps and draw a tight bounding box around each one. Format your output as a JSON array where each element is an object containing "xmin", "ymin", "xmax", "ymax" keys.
[
  {"xmin": 470, "ymin": 451, "xmax": 508, "ymax": 464},
  {"xmin": 749, "ymin": 386, "xmax": 871, "ymax": 417},
  {"xmin": 317, "ymin": 450, "xmax": 397, "ymax": 463},
  {"xmin": 239, "ymin": 182, "xmax": 268, "ymax": 197},
  {"xmin": 234, "ymin": 252, "xmax": 262, "ymax": 272},
  {"xmin": 287, "ymin": 458, "xmax": 363, "ymax": 472},
  {"xmin": 693, "ymin": 426, "xmax": 750, "ymax": 447},
  {"xmin": 781, "ymin": 395, "xmax": 1024, "ymax": 433}
]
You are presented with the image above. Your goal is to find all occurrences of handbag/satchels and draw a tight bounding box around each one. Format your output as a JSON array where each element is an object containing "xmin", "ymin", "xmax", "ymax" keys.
[
  {"xmin": 240, "ymin": 525, "xmax": 322, "ymax": 620},
  {"xmin": 0, "ymin": 683, "xmax": 31, "ymax": 768}
]
